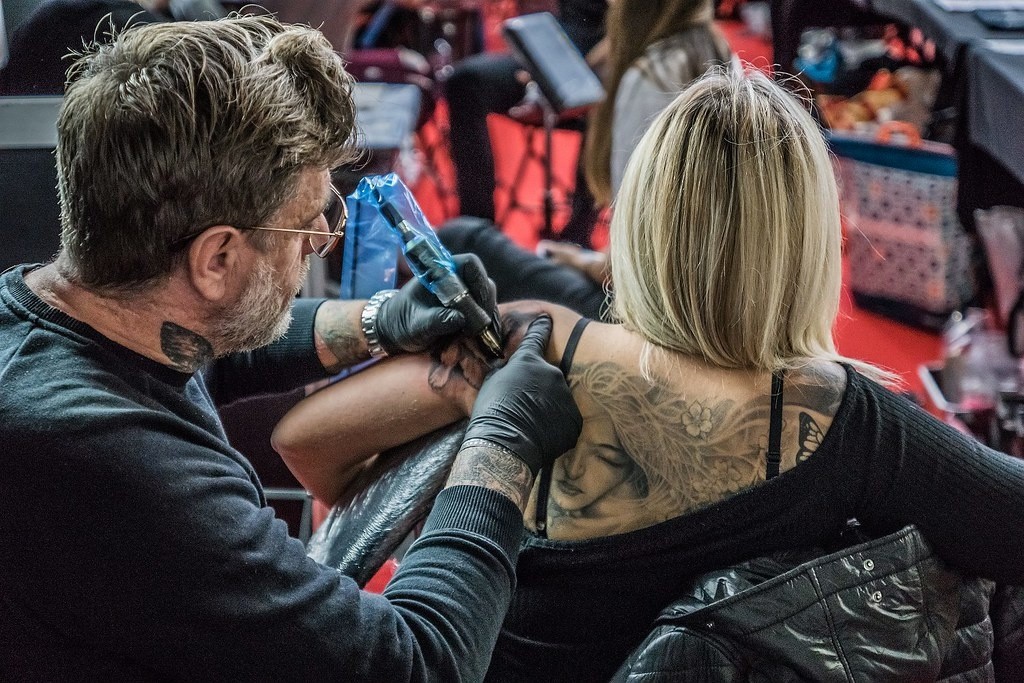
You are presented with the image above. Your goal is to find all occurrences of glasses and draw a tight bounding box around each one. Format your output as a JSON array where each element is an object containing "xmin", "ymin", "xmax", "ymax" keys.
[{"xmin": 169, "ymin": 183, "xmax": 348, "ymax": 258}]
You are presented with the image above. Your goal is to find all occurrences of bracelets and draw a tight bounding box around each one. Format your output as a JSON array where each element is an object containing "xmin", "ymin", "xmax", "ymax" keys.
[{"xmin": 361, "ymin": 289, "xmax": 399, "ymax": 357}]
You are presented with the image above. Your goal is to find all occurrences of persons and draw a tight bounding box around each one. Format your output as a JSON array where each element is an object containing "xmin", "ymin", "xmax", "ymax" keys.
[
  {"xmin": 272, "ymin": 63, "xmax": 1024, "ymax": 683},
  {"xmin": 430, "ymin": 0, "xmax": 739, "ymax": 319},
  {"xmin": 0, "ymin": 4, "xmax": 581, "ymax": 683}
]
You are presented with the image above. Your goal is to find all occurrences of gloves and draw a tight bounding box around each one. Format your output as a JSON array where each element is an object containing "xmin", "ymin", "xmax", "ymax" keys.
[
  {"xmin": 458, "ymin": 312, "xmax": 583, "ymax": 487},
  {"xmin": 376, "ymin": 253, "xmax": 496, "ymax": 352}
]
